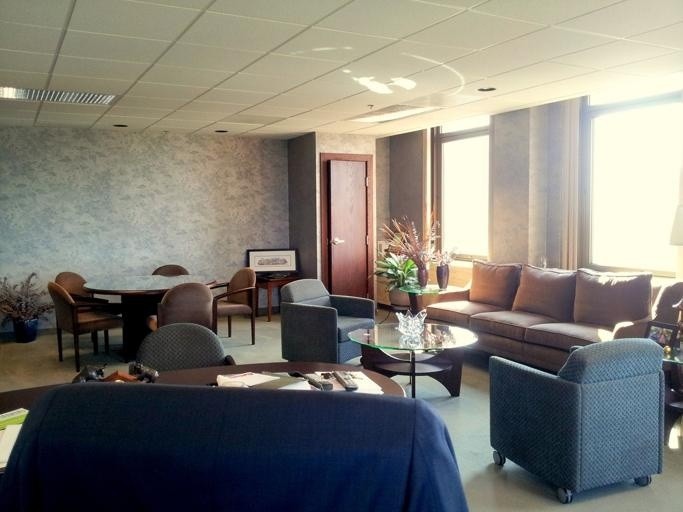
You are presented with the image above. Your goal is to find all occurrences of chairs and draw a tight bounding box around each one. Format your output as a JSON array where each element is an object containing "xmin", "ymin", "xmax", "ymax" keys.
[
  {"xmin": 488, "ymin": 338, "xmax": 665, "ymax": 504},
  {"xmin": 47, "ymin": 264, "xmax": 259, "ymax": 373},
  {"xmin": 279, "ymin": 278, "xmax": 376, "ymax": 362},
  {"xmin": 0, "ymin": 382, "xmax": 469, "ymax": 512}
]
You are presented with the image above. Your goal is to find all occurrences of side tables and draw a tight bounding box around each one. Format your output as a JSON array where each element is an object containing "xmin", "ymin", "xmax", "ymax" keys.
[
  {"xmin": 399, "ymin": 283, "xmax": 466, "ymax": 316},
  {"xmin": 663, "ymin": 344, "xmax": 683, "ymax": 409}
]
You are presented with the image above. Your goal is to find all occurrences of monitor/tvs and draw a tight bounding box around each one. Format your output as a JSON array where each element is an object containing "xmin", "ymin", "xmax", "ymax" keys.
[{"xmin": 246, "ymin": 248, "xmax": 298, "ymax": 279}]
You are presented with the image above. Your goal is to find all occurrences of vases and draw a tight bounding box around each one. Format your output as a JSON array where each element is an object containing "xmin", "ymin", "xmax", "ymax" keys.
[
  {"xmin": 419, "ymin": 267, "xmax": 427, "ymax": 287},
  {"xmin": 436, "ymin": 265, "xmax": 450, "ymax": 286}
]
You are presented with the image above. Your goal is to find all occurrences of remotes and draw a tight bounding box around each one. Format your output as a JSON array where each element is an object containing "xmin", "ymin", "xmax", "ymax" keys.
[
  {"xmin": 303, "ymin": 373, "xmax": 333, "ymax": 390},
  {"xmin": 334, "ymin": 370, "xmax": 358, "ymax": 391}
]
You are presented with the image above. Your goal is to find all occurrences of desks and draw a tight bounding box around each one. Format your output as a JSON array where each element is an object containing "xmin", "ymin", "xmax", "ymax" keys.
[
  {"xmin": 0, "ymin": 360, "xmax": 406, "ymax": 475},
  {"xmin": 257, "ymin": 276, "xmax": 299, "ymax": 322}
]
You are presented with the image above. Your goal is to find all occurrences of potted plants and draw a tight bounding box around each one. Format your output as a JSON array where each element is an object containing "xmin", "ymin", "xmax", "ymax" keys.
[
  {"xmin": 367, "ymin": 251, "xmax": 418, "ymax": 307},
  {"xmin": 0, "ymin": 270, "xmax": 54, "ymax": 342}
]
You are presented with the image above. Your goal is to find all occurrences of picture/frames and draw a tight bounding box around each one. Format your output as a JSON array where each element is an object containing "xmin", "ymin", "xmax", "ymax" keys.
[{"xmin": 246, "ymin": 248, "xmax": 298, "ymax": 274}]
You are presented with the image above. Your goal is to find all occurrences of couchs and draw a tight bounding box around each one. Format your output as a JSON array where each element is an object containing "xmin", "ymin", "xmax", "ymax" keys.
[{"xmin": 425, "ymin": 260, "xmax": 683, "ymax": 371}]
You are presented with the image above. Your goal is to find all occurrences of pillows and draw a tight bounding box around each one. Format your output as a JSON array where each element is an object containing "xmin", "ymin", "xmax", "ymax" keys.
[{"xmin": 468, "ymin": 262, "xmax": 653, "ymax": 325}]
